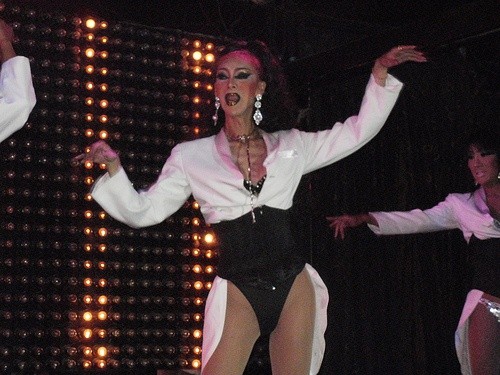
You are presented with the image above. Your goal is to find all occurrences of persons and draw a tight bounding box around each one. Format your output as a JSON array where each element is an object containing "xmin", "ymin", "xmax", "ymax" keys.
[
  {"xmin": 0, "ymin": 20, "xmax": 37, "ymax": 144},
  {"xmin": 72, "ymin": 37, "xmax": 430, "ymax": 375},
  {"xmin": 324, "ymin": 124, "xmax": 500, "ymax": 374}
]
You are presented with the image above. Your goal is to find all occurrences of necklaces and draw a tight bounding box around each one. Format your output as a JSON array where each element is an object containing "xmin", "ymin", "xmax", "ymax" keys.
[{"xmin": 224, "ymin": 127, "xmax": 260, "ymax": 225}]
